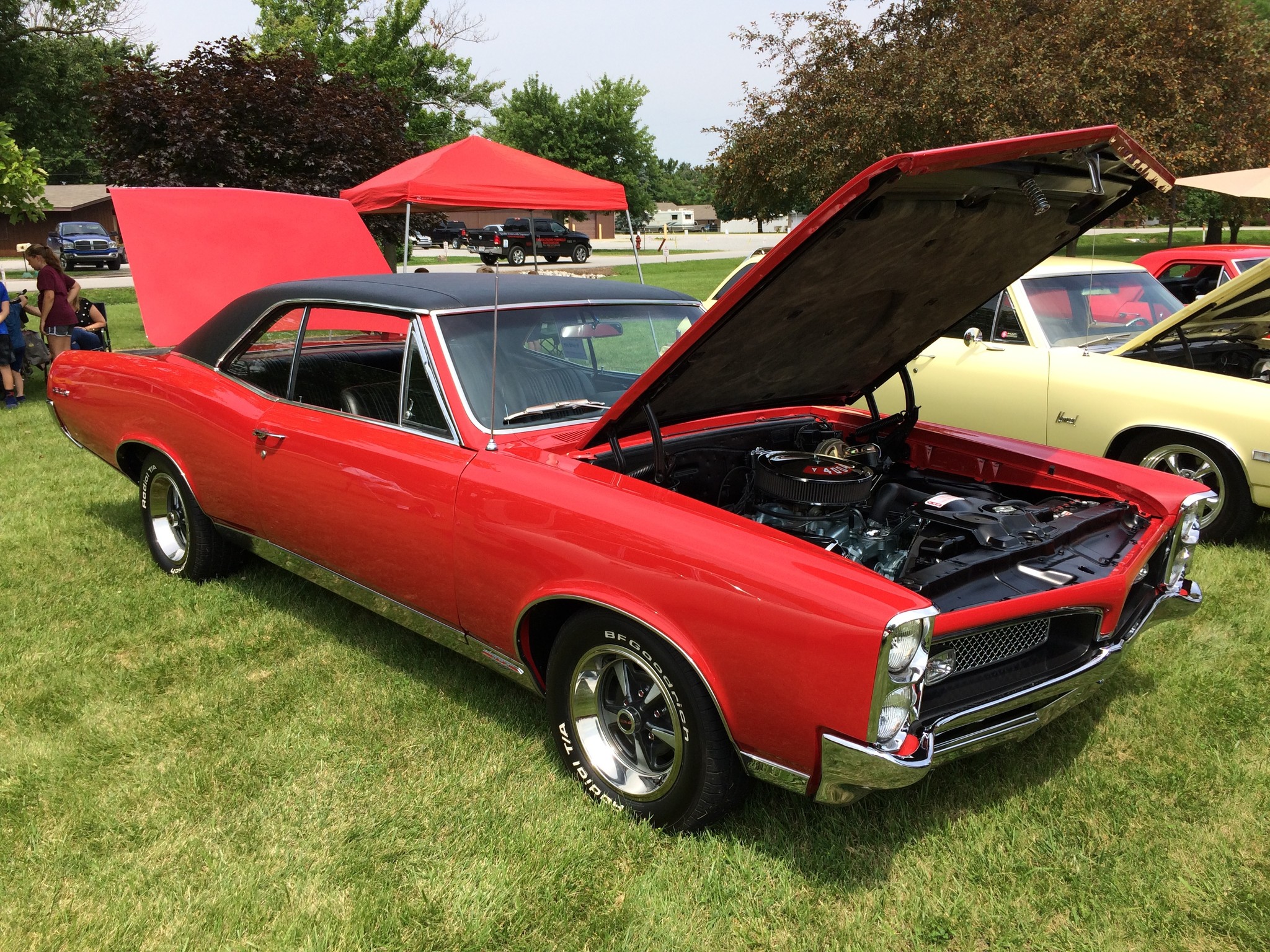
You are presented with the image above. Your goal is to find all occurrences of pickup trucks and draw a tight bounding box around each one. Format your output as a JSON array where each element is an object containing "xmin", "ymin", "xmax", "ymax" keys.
[
  {"xmin": 418, "ymin": 220, "xmax": 485, "ymax": 250},
  {"xmin": 689, "ymin": 221, "xmax": 706, "ymax": 233},
  {"xmin": 467, "ymin": 217, "xmax": 593, "ymax": 267}
]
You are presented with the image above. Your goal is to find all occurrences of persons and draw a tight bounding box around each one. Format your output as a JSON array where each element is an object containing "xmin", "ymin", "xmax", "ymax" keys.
[{"xmin": 0, "ymin": 244, "xmax": 106, "ymax": 409}]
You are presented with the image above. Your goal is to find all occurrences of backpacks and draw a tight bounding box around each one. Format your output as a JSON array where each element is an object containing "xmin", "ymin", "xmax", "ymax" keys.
[{"xmin": 21, "ymin": 330, "xmax": 51, "ymax": 365}]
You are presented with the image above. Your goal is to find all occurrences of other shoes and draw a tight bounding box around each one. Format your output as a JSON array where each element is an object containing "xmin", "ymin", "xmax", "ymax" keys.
[
  {"xmin": 16, "ymin": 396, "xmax": 26, "ymax": 402},
  {"xmin": 5, "ymin": 396, "xmax": 18, "ymax": 408}
]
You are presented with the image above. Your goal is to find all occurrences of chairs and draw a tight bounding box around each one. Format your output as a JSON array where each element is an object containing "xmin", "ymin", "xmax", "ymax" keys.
[
  {"xmin": 504, "ymin": 366, "xmax": 608, "ymax": 437},
  {"xmin": 338, "ymin": 376, "xmax": 488, "ymax": 443},
  {"xmin": 41, "ymin": 301, "xmax": 112, "ymax": 385}
]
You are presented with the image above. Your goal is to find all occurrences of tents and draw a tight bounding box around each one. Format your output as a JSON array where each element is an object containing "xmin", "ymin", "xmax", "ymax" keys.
[{"xmin": 339, "ymin": 135, "xmax": 661, "ymax": 359}]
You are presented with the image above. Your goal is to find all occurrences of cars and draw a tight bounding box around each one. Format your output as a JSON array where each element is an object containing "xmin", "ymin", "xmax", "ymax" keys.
[
  {"xmin": 993, "ymin": 243, "xmax": 1269, "ymax": 336},
  {"xmin": 45, "ymin": 121, "xmax": 1224, "ymax": 837},
  {"xmin": 408, "ymin": 228, "xmax": 432, "ymax": 250},
  {"xmin": 483, "ymin": 224, "xmax": 505, "ymax": 232},
  {"xmin": 656, "ymin": 247, "xmax": 1270, "ymax": 540},
  {"xmin": 47, "ymin": 221, "xmax": 122, "ymax": 271}
]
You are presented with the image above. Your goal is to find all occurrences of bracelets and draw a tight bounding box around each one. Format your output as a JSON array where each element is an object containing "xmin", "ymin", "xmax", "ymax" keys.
[{"xmin": 85, "ymin": 326, "xmax": 95, "ymax": 331}]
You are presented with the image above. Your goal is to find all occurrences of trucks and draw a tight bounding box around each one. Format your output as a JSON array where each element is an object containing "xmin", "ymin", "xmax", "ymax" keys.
[{"xmin": 636, "ymin": 208, "xmax": 696, "ymax": 233}]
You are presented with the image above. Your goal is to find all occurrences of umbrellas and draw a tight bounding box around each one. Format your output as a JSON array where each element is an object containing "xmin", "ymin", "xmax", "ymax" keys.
[{"xmin": 1174, "ymin": 167, "xmax": 1270, "ymax": 199}]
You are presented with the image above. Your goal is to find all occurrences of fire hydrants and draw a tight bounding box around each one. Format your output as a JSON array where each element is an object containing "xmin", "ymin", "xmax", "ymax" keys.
[{"xmin": 635, "ymin": 234, "xmax": 641, "ymax": 249}]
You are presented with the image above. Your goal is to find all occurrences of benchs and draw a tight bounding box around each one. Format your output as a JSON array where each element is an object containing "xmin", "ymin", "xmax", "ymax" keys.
[{"xmin": 241, "ymin": 342, "xmax": 499, "ymax": 410}]
